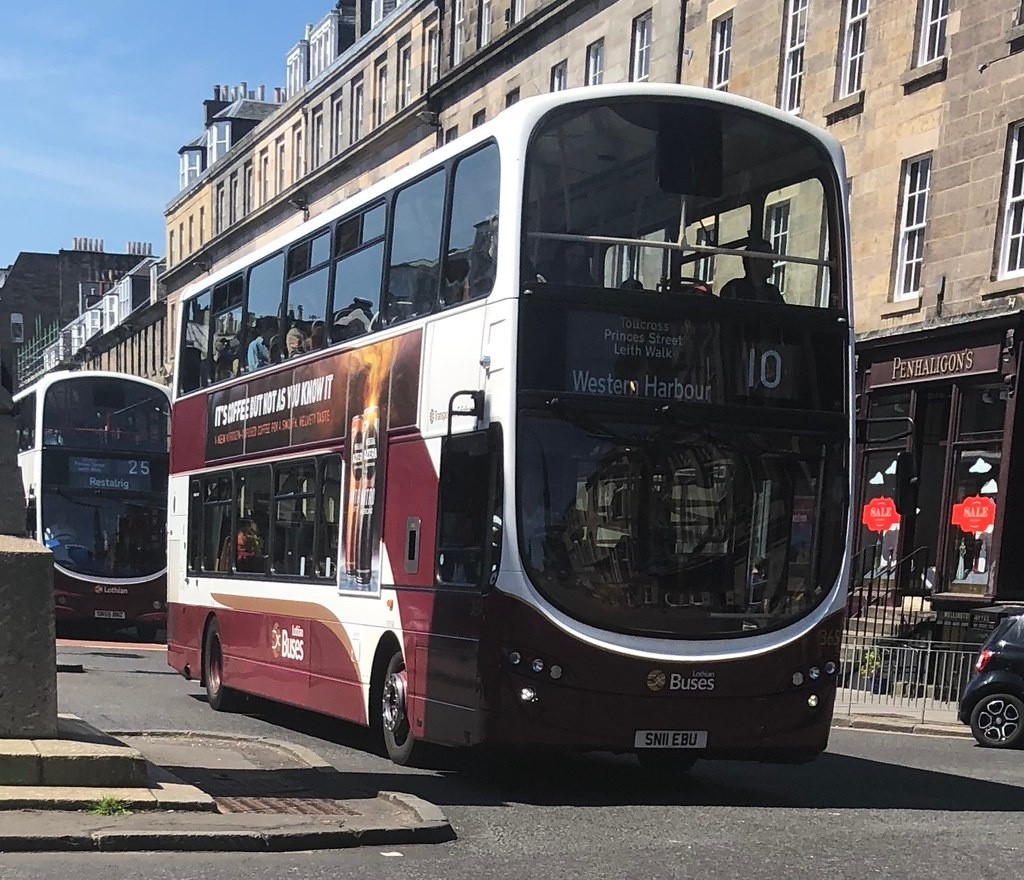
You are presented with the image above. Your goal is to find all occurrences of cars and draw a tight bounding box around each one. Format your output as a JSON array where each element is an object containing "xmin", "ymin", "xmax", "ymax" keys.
[{"xmin": 958, "ymin": 613, "xmax": 1024, "ymax": 748}]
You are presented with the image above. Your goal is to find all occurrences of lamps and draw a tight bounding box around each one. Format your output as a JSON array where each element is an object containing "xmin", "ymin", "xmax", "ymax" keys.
[
  {"xmin": 193, "ymin": 261, "xmax": 210, "ymax": 274},
  {"xmin": 288, "ymin": 199, "xmax": 308, "ymax": 221},
  {"xmin": 415, "ymin": 110, "xmax": 442, "ymax": 132}
]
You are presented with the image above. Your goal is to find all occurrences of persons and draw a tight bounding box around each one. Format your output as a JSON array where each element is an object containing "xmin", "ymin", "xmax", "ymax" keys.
[
  {"xmin": 237, "ymin": 513, "xmax": 269, "ymax": 559},
  {"xmin": 198, "ymin": 297, "xmax": 326, "ymax": 387},
  {"xmin": 719, "ymin": 238, "xmax": 786, "ymax": 302}
]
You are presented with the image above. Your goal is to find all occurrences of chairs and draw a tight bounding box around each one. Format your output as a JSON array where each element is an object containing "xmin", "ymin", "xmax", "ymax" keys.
[
  {"xmin": 310, "ymin": 319, "xmax": 324, "ymax": 349},
  {"xmin": 268, "ymin": 334, "xmax": 282, "ymax": 363}
]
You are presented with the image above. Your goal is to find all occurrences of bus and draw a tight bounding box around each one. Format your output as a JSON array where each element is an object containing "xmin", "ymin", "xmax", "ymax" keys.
[
  {"xmin": 167, "ymin": 81, "xmax": 917, "ymax": 766},
  {"xmin": 12, "ymin": 370, "xmax": 173, "ymax": 644}
]
